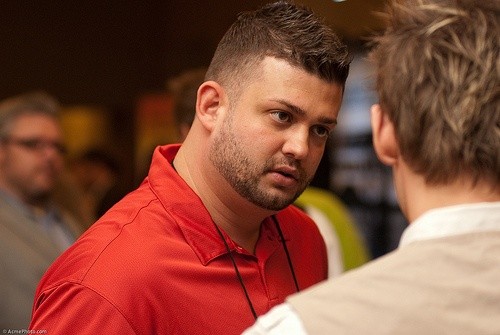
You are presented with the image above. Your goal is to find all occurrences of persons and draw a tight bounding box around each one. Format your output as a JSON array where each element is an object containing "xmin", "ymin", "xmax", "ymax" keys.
[
  {"xmin": 0, "ymin": 94, "xmax": 81, "ymax": 335},
  {"xmin": 241, "ymin": 1, "xmax": 499, "ymax": 335},
  {"xmin": 169, "ymin": 69, "xmax": 372, "ymax": 281},
  {"xmin": 44, "ymin": 107, "xmax": 117, "ymax": 239},
  {"xmin": 27, "ymin": 0, "xmax": 353, "ymax": 335}
]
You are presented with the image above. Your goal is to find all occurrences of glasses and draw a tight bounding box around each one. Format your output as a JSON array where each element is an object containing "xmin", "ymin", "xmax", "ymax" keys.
[{"xmin": 6, "ymin": 137, "xmax": 66, "ymax": 154}]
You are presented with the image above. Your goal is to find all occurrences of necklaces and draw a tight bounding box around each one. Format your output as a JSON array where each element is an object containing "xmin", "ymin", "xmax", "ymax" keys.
[{"xmin": 169, "ymin": 158, "xmax": 301, "ymax": 323}]
